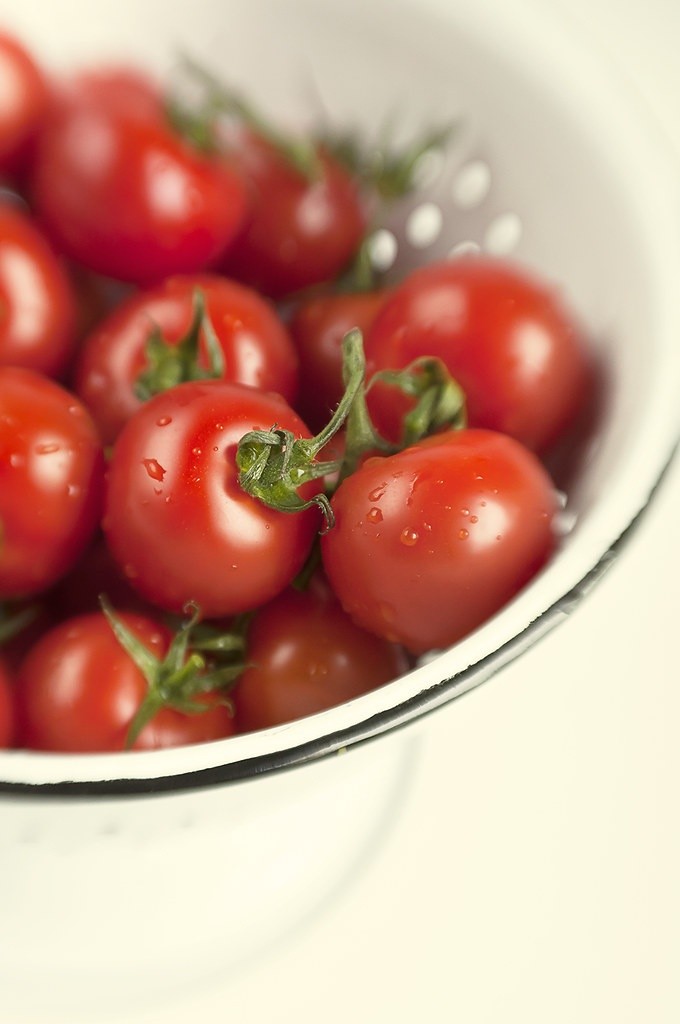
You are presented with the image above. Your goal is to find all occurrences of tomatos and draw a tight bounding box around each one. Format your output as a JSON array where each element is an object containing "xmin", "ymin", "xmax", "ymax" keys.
[{"xmin": 0, "ymin": 32, "xmax": 586, "ymax": 752}]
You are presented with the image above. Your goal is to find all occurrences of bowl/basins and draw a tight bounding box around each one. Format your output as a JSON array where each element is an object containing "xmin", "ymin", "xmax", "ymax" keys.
[{"xmin": 0, "ymin": 0, "xmax": 680, "ymax": 785}]
